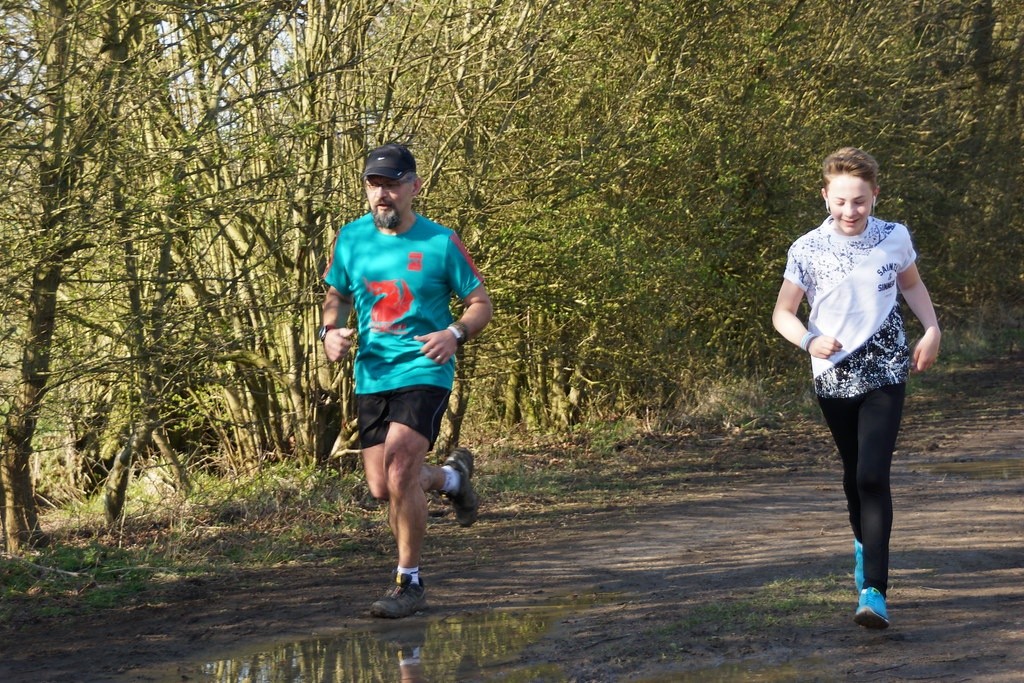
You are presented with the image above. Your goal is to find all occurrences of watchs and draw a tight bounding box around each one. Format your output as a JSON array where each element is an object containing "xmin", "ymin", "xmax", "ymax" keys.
[{"xmin": 319, "ymin": 325, "xmax": 335, "ymax": 341}]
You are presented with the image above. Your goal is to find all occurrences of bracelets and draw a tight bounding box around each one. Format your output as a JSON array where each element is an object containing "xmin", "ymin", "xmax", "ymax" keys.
[
  {"xmin": 800, "ymin": 333, "xmax": 815, "ymax": 351},
  {"xmin": 449, "ymin": 321, "xmax": 468, "ymax": 347}
]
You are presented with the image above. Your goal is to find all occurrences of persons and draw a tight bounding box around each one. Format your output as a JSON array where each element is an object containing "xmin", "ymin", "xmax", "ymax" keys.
[
  {"xmin": 322, "ymin": 145, "xmax": 493, "ymax": 617},
  {"xmin": 772, "ymin": 147, "xmax": 941, "ymax": 629}
]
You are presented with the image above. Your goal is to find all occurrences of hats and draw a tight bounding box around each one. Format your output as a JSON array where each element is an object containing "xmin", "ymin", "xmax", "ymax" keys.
[{"xmin": 362, "ymin": 143, "xmax": 416, "ymax": 179}]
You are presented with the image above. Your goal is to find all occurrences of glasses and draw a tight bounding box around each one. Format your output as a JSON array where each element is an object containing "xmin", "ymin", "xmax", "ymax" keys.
[{"xmin": 364, "ymin": 175, "xmax": 417, "ymax": 190}]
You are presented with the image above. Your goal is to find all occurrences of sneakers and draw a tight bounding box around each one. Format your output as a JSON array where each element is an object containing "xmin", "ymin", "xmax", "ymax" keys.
[
  {"xmin": 370, "ymin": 569, "xmax": 429, "ymax": 619},
  {"xmin": 853, "ymin": 587, "xmax": 890, "ymax": 630},
  {"xmin": 854, "ymin": 538, "xmax": 865, "ymax": 593},
  {"xmin": 438, "ymin": 448, "xmax": 478, "ymax": 527}
]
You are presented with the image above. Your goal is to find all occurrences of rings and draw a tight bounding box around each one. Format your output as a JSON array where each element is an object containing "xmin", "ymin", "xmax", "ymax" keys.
[{"xmin": 437, "ymin": 356, "xmax": 443, "ymax": 361}]
